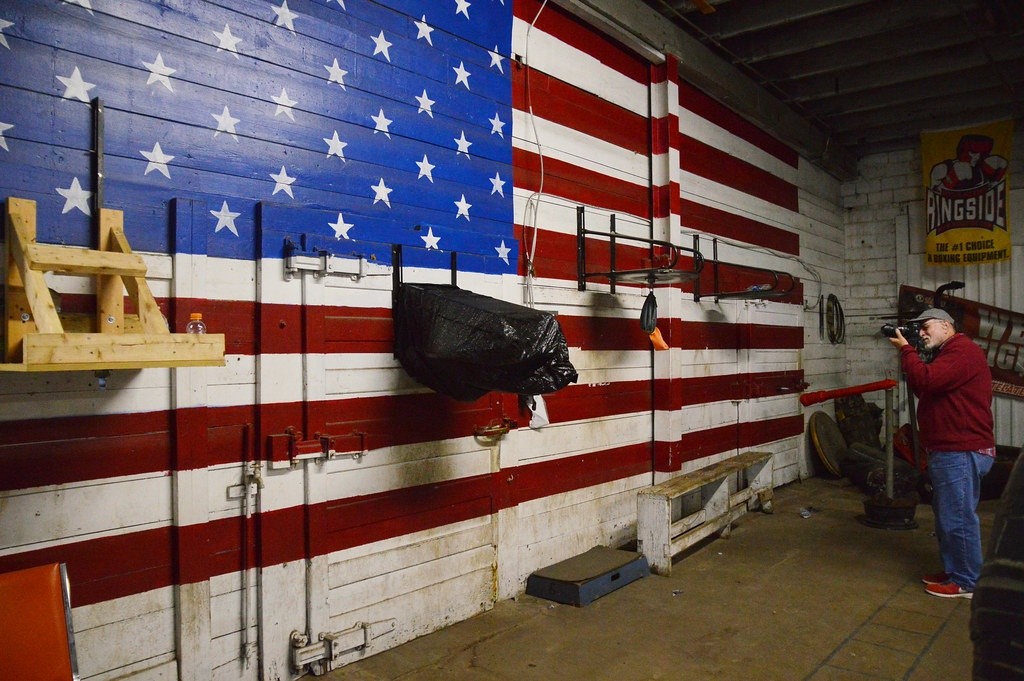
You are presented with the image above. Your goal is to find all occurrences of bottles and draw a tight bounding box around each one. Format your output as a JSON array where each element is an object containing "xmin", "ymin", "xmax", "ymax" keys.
[{"xmin": 186, "ymin": 313, "xmax": 206, "ymax": 334}]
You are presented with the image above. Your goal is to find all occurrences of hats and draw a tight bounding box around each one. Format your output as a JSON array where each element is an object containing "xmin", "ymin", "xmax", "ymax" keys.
[{"xmin": 906, "ymin": 308, "xmax": 955, "ymax": 326}]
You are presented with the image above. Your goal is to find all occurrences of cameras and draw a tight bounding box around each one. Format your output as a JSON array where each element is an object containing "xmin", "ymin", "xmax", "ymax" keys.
[{"xmin": 880, "ymin": 323, "xmax": 920, "ymax": 345}]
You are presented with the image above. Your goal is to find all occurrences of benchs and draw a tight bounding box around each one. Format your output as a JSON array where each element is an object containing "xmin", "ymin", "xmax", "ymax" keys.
[{"xmin": 637, "ymin": 451, "xmax": 776, "ymax": 577}]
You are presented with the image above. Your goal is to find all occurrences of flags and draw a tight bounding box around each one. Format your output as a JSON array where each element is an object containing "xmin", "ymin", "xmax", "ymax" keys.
[{"xmin": 919, "ymin": 115, "xmax": 1016, "ymax": 268}]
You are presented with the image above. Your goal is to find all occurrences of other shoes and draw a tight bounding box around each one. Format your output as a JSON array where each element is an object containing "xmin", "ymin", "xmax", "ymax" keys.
[
  {"xmin": 921, "ymin": 573, "xmax": 952, "ymax": 584},
  {"xmin": 925, "ymin": 581, "xmax": 974, "ymax": 599}
]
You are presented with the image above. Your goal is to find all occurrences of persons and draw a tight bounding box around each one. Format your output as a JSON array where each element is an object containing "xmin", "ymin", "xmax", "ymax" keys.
[{"xmin": 890, "ymin": 308, "xmax": 996, "ymax": 598}]
